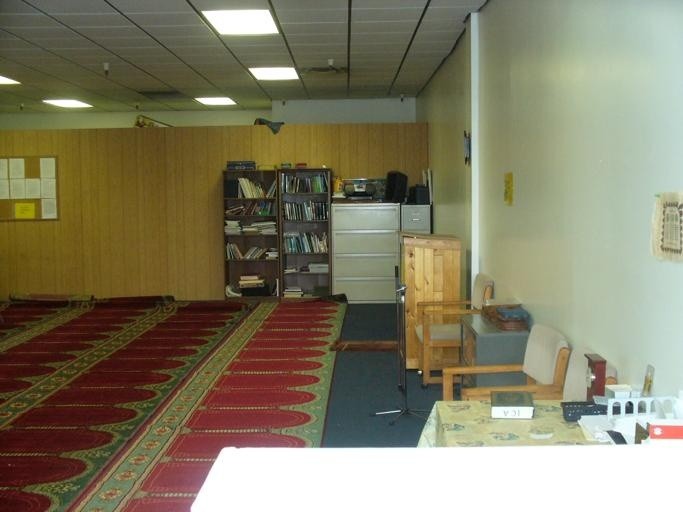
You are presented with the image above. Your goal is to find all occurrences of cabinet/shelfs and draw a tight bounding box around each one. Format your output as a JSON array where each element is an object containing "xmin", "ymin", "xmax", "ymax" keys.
[
  {"xmin": 454, "ymin": 311, "xmax": 531, "ymax": 389},
  {"xmin": 220, "ymin": 168, "xmax": 279, "ymax": 303},
  {"xmin": 328, "ymin": 202, "xmax": 400, "ymax": 304},
  {"xmin": 399, "ymin": 204, "xmax": 430, "ymax": 235},
  {"xmin": 276, "ymin": 168, "xmax": 332, "ymax": 302}
]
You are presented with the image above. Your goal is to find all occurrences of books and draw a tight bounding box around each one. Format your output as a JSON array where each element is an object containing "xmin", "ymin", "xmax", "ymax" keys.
[
  {"xmin": 576, "ymin": 412, "xmax": 683, "ymax": 444},
  {"xmin": 223, "ymin": 160, "xmax": 329, "ymax": 298},
  {"xmin": 490, "ymin": 390, "xmax": 535, "ymax": 420}
]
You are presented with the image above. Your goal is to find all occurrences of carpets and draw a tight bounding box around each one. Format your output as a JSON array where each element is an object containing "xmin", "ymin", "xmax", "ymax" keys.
[{"xmin": 0, "ymin": 286, "xmax": 345, "ymax": 512}]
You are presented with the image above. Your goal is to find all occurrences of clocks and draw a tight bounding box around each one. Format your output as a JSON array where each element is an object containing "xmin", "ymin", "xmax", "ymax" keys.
[{"xmin": 583, "ymin": 353, "xmax": 606, "ymax": 403}]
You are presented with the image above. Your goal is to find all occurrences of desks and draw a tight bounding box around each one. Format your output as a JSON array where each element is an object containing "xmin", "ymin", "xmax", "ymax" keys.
[{"xmin": 411, "ymin": 396, "xmax": 682, "ymax": 447}]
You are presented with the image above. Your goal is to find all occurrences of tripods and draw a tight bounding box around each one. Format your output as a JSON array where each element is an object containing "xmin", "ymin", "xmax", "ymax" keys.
[{"xmin": 372, "ymin": 287, "xmax": 432, "ymax": 426}]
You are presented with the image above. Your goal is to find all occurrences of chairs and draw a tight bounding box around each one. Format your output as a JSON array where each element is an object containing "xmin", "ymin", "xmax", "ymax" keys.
[
  {"xmin": 415, "ymin": 274, "xmax": 493, "ymax": 387},
  {"xmin": 441, "ymin": 322, "xmax": 570, "ymax": 402}
]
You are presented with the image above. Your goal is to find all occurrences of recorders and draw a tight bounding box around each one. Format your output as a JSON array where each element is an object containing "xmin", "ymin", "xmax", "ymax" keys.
[{"xmin": 344, "ymin": 182, "xmax": 376, "ymax": 196}]
